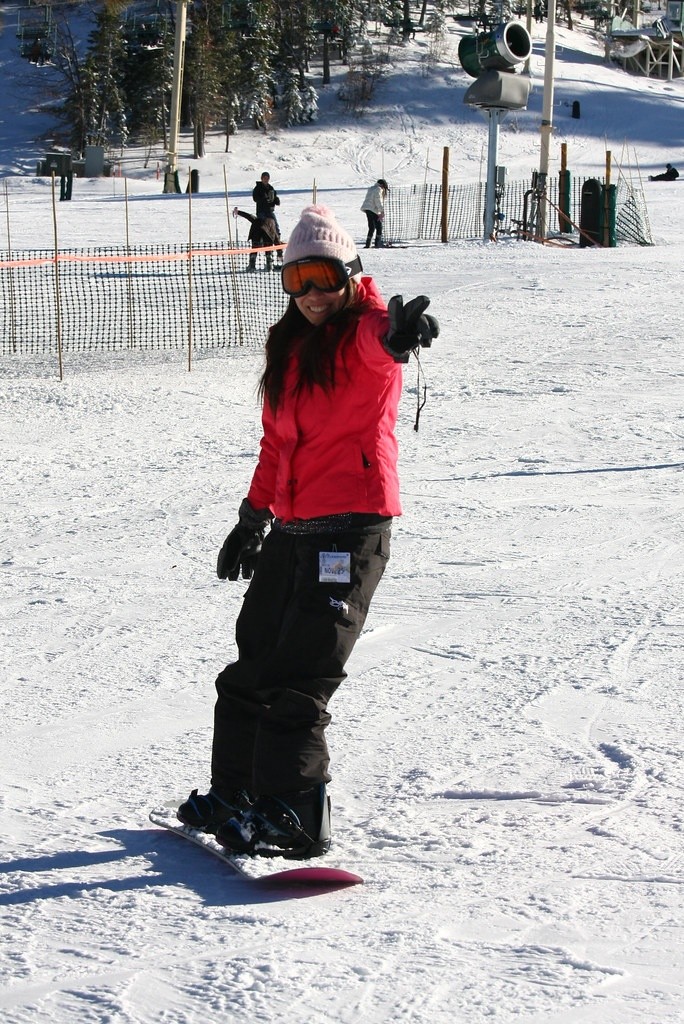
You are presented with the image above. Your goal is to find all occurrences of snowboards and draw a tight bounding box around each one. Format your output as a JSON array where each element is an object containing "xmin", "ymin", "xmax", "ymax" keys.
[{"xmin": 149, "ymin": 799, "xmax": 364, "ymax": 887}]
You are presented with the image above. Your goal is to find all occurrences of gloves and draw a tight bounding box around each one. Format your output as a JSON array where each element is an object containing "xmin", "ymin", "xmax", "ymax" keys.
[
  {"xmin": 382, "ymin": 294, "xmax": 440, "ymax": 359},
  {"xmin": 216, "ymin": 498, "xmax": 275, "ymax": 581}
]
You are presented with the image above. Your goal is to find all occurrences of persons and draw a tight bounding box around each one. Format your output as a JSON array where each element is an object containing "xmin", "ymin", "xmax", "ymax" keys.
[
  {"xmin": 235, "ymin": 171, "xmax": 391, "ymax": 272},
  {"xmin": 176, "ymin": 206, "xmax": 440, "ymax": 859},
  {"xmin": 649, "ymin": 164, "xmax": 679, "ymax": 181}
]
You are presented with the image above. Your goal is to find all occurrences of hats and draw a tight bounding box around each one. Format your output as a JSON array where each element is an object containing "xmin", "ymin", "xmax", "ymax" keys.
[{"xmin": 282, "ymin": 204, "xmax": 362, "ymax": 282}]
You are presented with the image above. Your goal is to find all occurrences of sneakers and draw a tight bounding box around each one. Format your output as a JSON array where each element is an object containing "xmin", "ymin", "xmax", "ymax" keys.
[
  {"xmin": 175, "ymin": 783, "xmax": 254, "ymax": 831},
  {"xmin": 216, "ymin": 784, "xmax": 332, "ymax": 858}
]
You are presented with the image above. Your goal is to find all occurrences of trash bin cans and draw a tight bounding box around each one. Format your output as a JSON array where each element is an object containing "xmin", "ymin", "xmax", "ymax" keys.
[
  {"xmin": 579, "ymin": 179, "xmax": 605, "ymax": 247},
  {"xmin": 191, "ymin": 169, "xmax": 199, "ymax": 192}
]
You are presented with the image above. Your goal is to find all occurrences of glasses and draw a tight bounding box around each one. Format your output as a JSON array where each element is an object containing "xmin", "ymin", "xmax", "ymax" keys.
[{"xmin": 281, "ymin": 254, "xmax": 363, "ymax": 298}]
